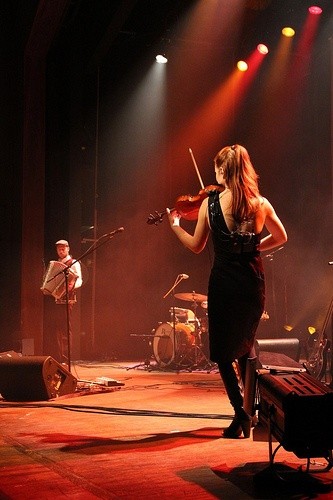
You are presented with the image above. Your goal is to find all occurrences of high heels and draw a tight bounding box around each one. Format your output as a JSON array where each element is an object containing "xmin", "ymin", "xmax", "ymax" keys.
[{"xmin": 219, "ymin": 406, "xmax": 257, "ymax": 438}]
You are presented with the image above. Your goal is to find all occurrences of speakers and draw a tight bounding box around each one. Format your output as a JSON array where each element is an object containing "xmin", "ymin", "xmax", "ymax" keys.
[
  {"xmin": 254, "ymin": 337, "xmax": 300, "ymax": 362},
  {"xmin": 0, "ymin": 351, "xmax": 78, "ymax": 402}
]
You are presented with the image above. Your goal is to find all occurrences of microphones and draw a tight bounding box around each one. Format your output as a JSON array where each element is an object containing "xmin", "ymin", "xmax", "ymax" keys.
[
  {"xmin": 103, "ymin": 227, "xmax": 124, "ymax": 237},
  {"xmin": 157, "ymin": 334, "xmax": 169, "ymax": 338},
  {"xmin": 179, "ymin": 274, "xmax": 189, "ymax": 279}
]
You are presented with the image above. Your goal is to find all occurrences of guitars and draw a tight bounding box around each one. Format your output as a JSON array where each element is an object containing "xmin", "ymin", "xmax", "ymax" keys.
[{"xmin": 305, "ymin": 300, "xmax": 333, "ymax": 382}]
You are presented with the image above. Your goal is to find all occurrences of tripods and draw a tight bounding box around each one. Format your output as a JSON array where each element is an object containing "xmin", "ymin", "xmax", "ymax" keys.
[{"xmin": 123, "ymin": 279, "xmax": 212, "ymax": 374}]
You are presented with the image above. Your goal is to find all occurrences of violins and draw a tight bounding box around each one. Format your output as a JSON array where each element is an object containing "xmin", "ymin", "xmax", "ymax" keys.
[{"xmin": 147, "ymin": 185, "xmax": 223, "ymax": 226}]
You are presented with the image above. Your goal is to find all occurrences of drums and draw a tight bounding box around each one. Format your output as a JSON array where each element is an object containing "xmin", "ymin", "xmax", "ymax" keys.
[
  {"xmin": 175, "ymin": 309, "xmax": 199, "ymax": 324},
  {"xmin": 200, "ymin": 302, "xmax": 209, "ymax": 333},
  {"xmin": 150, "ymin": 323, "xmax": 198, "ymax": 361}
]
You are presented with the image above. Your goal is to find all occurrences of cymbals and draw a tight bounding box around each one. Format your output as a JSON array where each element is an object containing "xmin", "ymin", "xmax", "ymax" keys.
[
  {"xmin": 173, "ymin": 292, "xmax": 209, "ymax": 301},
  {"xmin": 169, "ymin": 309, "xmax": 187, "ymax": 315}
]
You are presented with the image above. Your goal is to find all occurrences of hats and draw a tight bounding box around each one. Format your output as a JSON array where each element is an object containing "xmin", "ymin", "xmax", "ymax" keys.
[{"xmin": 56, "ymin": 239, "xmax": 69, "ymax": 246}]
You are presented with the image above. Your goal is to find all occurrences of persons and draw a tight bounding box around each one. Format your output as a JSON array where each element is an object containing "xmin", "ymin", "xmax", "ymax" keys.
[
  {"xmin": 166, "ymin": 144, "xmax": 288, "ymax": 438},
  {"xmin": 40, "ymin": 240, "xmax": 82, "ymax": 367}
]
